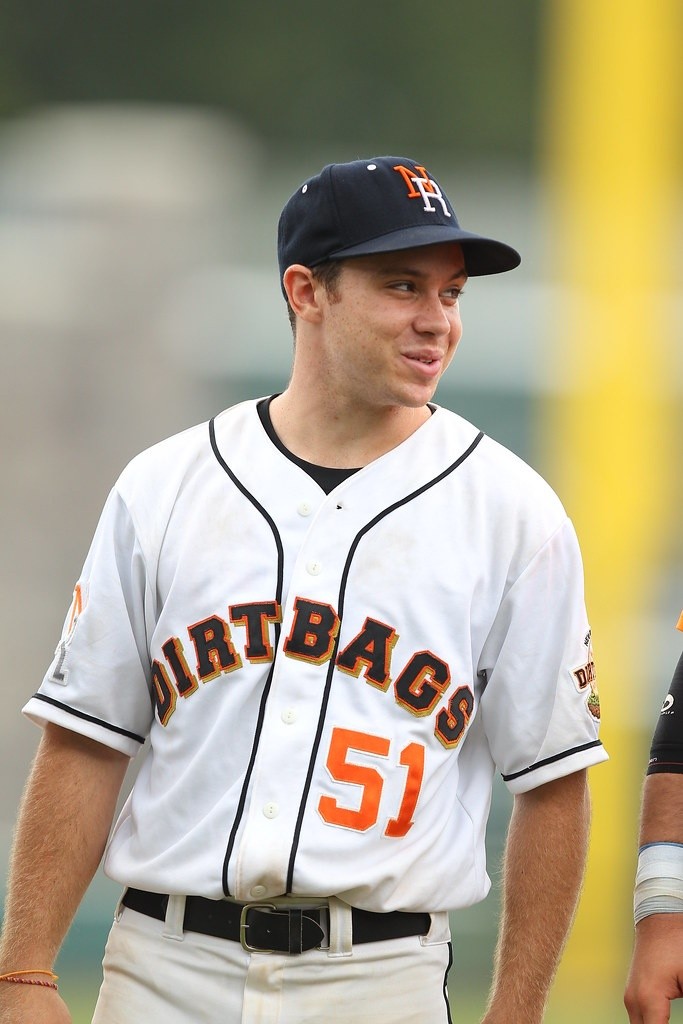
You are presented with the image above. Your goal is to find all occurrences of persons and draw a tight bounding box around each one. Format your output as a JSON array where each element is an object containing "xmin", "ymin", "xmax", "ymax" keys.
[
  {"xmin": 623, "ymin": 604, "xmax": 683, "ymax": 1024},
  {"xmin": 2, "ymin": 156, "xmax": 612, "ymax": 1024}
]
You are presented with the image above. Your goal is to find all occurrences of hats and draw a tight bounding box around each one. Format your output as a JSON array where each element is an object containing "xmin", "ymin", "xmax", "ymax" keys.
[{"xmin": 279, "ymin": 156, "xmax": 521, "ymax": 277}]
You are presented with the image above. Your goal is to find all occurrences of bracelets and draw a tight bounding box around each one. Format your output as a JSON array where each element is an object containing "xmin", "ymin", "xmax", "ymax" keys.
[
  {"xmin": 2, "ymin": 966, "xmax": 61, "ymax": 994},
  {"xmin": 631, "ymin": 841, "xmax": 683, "ymax": 927}
]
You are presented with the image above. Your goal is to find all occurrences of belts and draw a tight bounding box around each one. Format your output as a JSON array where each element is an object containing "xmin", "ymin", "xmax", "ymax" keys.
[{"xmin": 120, "ymin": 886, "xmax": 432, "ymax": 955}]
